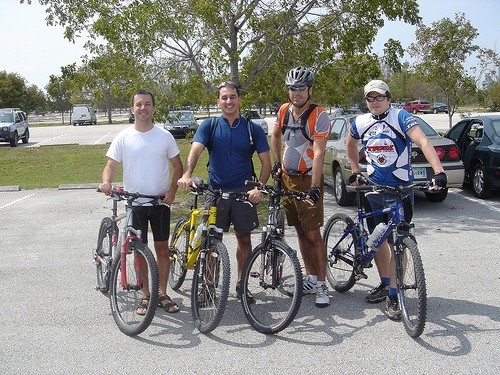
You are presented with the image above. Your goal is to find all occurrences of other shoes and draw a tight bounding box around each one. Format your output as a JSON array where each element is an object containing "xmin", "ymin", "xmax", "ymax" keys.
[
  {"xmin": 196, "ymin": 288, "xmax": 215, "ymax": 306},
  {"xmin": 236, "ymin": 284, "xmax": 253, "ymax": 302}
]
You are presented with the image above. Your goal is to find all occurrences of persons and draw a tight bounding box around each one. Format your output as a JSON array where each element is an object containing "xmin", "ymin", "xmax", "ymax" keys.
[
  {"xmin": 177, "ymin": 82, "xmax": 271, "ymax": 306},
  {"xmin": 98, "ymin": 89, "xmax": 183, "ymax": 315},
  {"xmin": 271, "ymin": 66, "xmax": 330, "ymax": 306},
  {"xmin": 346, "ymin": 80, "xmax": 448, "ymax": 320}
]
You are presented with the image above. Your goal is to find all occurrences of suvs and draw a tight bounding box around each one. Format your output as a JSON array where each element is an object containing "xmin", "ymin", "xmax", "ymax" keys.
[
  {"xmin": 271, "ymin": 102, "xmax": 283, "ymax": 115},
  {"xmin": 0, "ymin": 108, "xmax": 30, "ymax": 147}
]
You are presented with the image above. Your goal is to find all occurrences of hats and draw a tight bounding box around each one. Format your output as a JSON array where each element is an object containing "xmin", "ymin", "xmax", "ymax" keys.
[{"xmin": 364, "ymin": 80, "xmax": 390, "ymax": 97}]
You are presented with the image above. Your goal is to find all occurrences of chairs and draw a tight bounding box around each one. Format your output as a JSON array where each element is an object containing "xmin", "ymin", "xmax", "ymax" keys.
[{"xmin": 462, "ymin": 129, "xmax": 483, "ymax": 167}]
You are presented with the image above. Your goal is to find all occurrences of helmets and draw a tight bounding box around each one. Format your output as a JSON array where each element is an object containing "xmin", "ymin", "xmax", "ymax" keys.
[{"xmin": 285, "ymin": 66, "xmax": 315, "ymax": 86}]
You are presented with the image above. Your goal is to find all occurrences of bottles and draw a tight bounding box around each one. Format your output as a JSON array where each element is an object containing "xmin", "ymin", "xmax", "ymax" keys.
[
  {"xmin": 191, "ymin": 223, "xmax": 205, "ymax": 248},
  {"xmin": 366, "ymin": 222, "xmax": 387, "ymax": 248}
]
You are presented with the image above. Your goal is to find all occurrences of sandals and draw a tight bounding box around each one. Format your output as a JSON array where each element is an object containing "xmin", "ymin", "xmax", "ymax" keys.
[
  {"xmin": 157, "ymin": 295, "xmax": 179, "ymax": 313},
  {"xmin": 136, "ymin": 296, "xmax": 150, "ymax": 315}
]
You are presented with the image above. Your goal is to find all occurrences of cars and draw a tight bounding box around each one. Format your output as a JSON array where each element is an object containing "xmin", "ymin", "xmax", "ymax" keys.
[
  {"xmin": 328, "ymin": 109, "xmax": 363, "ymax": 120},
  {"xmin": 325, "ymin": 113, "xmax": 464, "ymax": 209},
  {"xmin": 164, "ymin": 110, "xmax": 198, "ymax": 139},
  {"xmin": 443, "ymin": 115, "xmax": 500, "ymax": 199},
  {"xmin": 240, "ymin": 109, "xmax": 268, "ymax": 136},
  {"xmin": 389, "ymin": 100, "xmax": 449, "ymax": 115}
]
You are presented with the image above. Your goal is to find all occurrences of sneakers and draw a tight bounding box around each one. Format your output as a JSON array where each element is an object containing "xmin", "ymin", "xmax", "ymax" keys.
[
  {"xmin": 316, "ymin": 285, "xmax": 330, "ymax": 307},
  {"xmin": 365, "ymin": 282, "xmax": 391, "ymax": 303},
  {"xmin": 386, "ymin": 294, "xmax": 402, "ymax": 319},
  {"xmin": 287, "ymin": 276, "xmax": 316, "ymax": 296}
]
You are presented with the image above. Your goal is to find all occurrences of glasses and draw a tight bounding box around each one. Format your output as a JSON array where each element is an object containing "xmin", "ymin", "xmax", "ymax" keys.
[
  {"xmin": 288, "ymin": 85, "xmax": 310, "ymax": 92},
  {"xmin": 218, "ymin": 81, "xmax": 238, "ymax": 90},
  {"xmin": 365, "ymin": 94, "xmax": 388, "ymax": 102}
]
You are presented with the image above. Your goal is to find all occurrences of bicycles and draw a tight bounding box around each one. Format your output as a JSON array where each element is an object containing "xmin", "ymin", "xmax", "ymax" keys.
[
  {"xmin": 168, "ymin": 176, "xmax": 258, "ymax": 335},
  {"xmin": 94, "ymin": 188, "xmax": 166, "ymax": 338},
  {"xmin": 323, "ymin": 182, "xmax": 436, "ymax": 338},
  {"xmin": 244, "ymin": 179, "xmax": 316, "ymax": 337}
]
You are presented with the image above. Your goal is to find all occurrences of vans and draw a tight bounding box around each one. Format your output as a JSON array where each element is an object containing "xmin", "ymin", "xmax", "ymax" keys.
[
  {"xmin": 71, "ymin": 103, "xmax": 97, "ymax": 125},
  {"xmin": 129, "ymin": 110, "xmax": 135, "ymax": 123}
]
